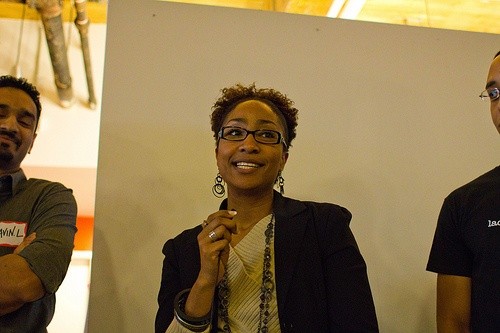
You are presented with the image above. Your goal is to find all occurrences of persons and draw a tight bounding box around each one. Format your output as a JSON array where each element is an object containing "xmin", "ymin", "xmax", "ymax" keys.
[
  {"xmin": 154, "ymin": 81, "xmax": 380, "ymax": 333},
  {"xmin": 0, "ymin": 74, "xmax": 77, "ymax": 331},
  {"xmin": 424, "ymin": 50, "xmax": 499, "ymax": 332}
]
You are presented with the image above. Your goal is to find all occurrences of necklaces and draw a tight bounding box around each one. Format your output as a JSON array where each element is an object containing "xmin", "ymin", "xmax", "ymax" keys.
[{"xmin": 216, "ymin": 210, "xmax": 274, "ymax": 332}]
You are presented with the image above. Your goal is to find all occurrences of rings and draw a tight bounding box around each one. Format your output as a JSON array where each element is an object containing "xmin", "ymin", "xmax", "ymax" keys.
[
  {"xmin": 208, "ymin": 230, "xmax": 218, "ymax": 241},
  {"xmin": 202, "ymin": 219, "xmax": 209, "ymax": 227}
]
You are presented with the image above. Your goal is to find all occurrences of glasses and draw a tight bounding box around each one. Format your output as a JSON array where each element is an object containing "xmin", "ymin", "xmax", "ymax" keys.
[
  {"xmin": 217, "ymin": 125, "xmax": 287, "ymax": 147},
  {"xmin": 479, "ymin": 86, "xmax": 500, "ymax": 102}
]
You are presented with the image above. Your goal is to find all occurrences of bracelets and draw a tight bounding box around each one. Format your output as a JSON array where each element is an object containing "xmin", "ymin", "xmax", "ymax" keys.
[{"xmin": 172, "ymin": 287, "xmax": 215, "ymax": 330}]
